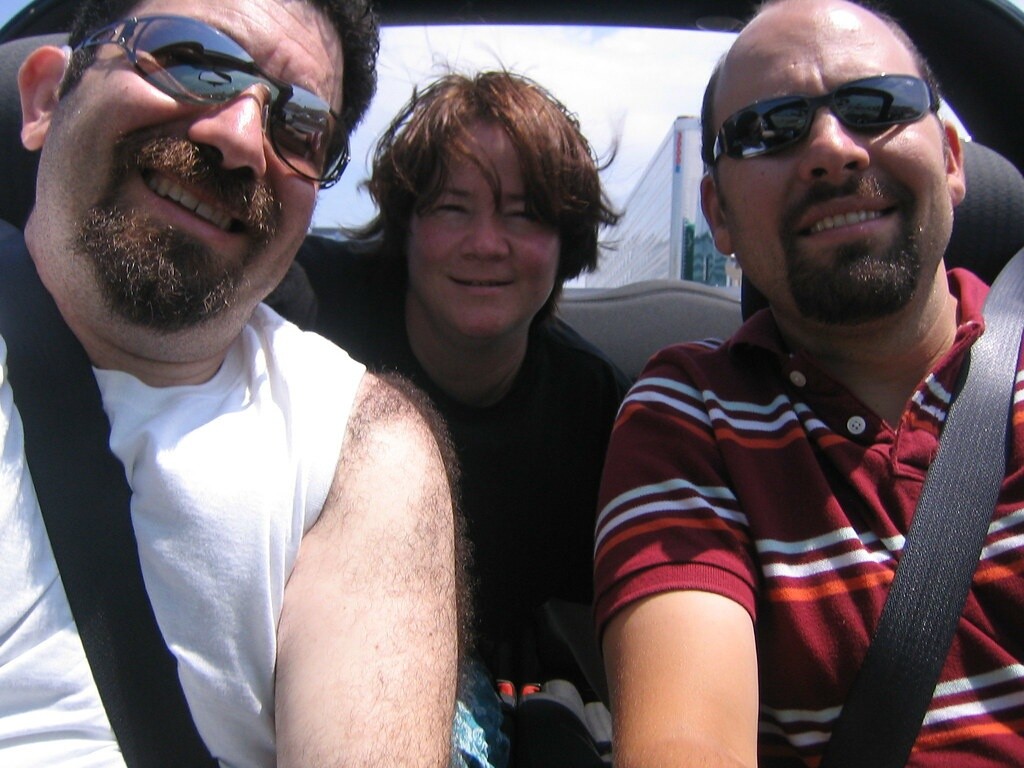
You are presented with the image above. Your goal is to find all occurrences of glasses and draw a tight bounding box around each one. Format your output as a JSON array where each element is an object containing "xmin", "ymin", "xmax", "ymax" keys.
[
  {"xmin": 710, "ymin": 73, "xmax": 935, "ymax": 174},
  {"xmin": 73, "ymin": 14, "xmax": 353, "ymax": 190}
]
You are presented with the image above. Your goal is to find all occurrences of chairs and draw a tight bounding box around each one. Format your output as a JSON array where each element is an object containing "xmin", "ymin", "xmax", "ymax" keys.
[{"xmin": 740, "ymin": 144, "xmax": 1024, "ymax": 321}]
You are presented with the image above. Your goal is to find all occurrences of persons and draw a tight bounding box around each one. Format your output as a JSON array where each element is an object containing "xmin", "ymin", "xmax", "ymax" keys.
[
  {"xmin": 262, "ymin": 66, "xmax": 630, "ymax": 766},
  {"xmin": 0, "ymin": 0, "xmax": 463, "ymax": 768},
  {"xmin": 593, "ymin": 1, "xmax": 1024, "ymax": 768}
]
